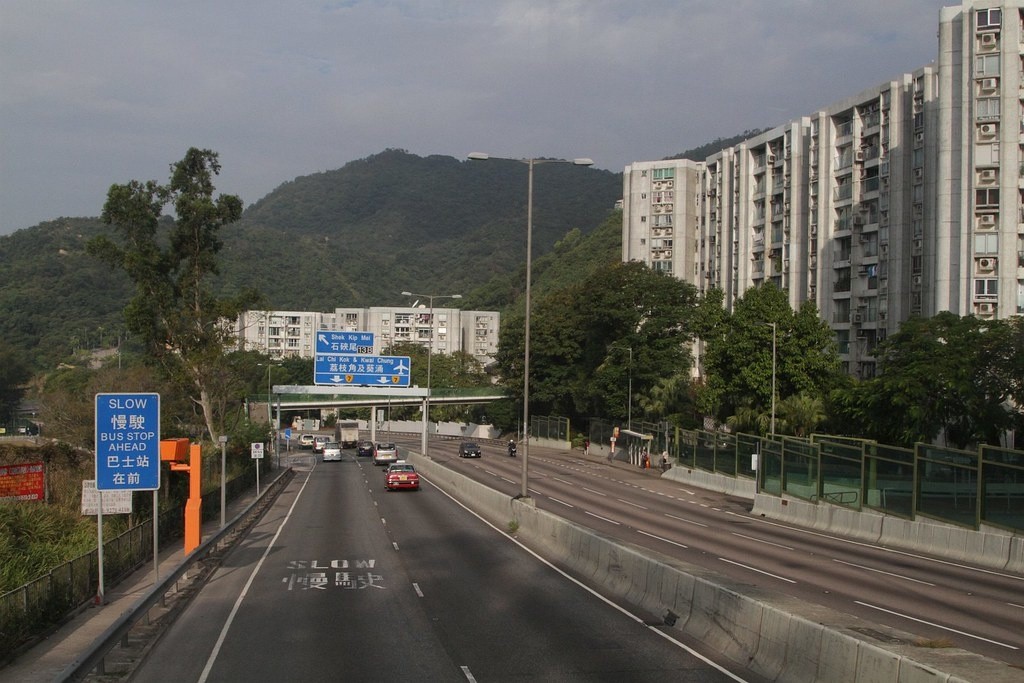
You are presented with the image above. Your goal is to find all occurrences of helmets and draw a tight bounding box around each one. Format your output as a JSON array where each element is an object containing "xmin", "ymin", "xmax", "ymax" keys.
[{"xmin": 510, "ymin": 439, "xmax": 513, "ymax": 443}]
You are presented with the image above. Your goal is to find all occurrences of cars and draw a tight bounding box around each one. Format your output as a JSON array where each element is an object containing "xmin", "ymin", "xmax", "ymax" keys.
[
  {"xmin": 356, "ymin": 441, "xmax": 375, "ymax": 456},
  {"xmin": 322, "ymin": 442, "xmax": 341, "ymax": 461},
  {"xmin": 17, "ymin": 426, "xmax": 29, "ymax": 435},
  {"xmin": 459, "ymin": 442, "xmax": 481, "ymax": 458},
  {"xmin": 383, "ymin": 459, "xmax": 419, "ymax": 491},
  {"xmin": 310, "ymin": 436, "xmax": 331, "ymax": 452}
]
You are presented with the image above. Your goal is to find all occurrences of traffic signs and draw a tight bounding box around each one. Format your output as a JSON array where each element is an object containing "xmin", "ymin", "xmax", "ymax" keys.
[{"xmin": 314, "ymin": 330, "xmax": 411, "ymax": 387}]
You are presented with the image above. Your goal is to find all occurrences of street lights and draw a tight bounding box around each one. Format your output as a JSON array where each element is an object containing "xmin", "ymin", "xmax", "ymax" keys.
[
  {"xmin": 767, "ymin": 322, "xmax": 776, "ymax": 439},
  {"xmin": 467, "ymin": 152, "xmax": 591, "ymax": 497},
  {"xmin": 624, "ymin": 348, "xmax": 632, "ymax": 432},
  {"xmin": 258, "ymin": 363, "xmax": 285, "ymax": 454},
  {"xmin": 219, "ymin": 436, "xmax": 232, "ymax": 529},
  {"xmin": 401, "ymin": 291, "xmax": 462, "ymax": 457}
]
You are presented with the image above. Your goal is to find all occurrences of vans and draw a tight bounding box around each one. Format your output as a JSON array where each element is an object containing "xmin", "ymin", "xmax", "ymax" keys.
[
  {"xmin": 373, "ymin": 441, "xmax": 399, "ymax": 465},
  {"xmin": 296, "ymin": 435, "xmax": 314, "ymax": 449}
]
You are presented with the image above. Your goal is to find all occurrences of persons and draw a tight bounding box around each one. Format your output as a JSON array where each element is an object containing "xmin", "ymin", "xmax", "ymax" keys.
[
  {"xmin": 662, "ymin": 449, "xmax": 669, "ymax": 463},
  {"xmin": 641, "ymin": 449, "xmax": 647, "ymax": 470},
  {"xmin": 583, "ymin": 439, "xmax": 590, "ymax": 455},
  {"xmin": 507, "ymin": 439, "xmax": 516, "ymax": 454}
]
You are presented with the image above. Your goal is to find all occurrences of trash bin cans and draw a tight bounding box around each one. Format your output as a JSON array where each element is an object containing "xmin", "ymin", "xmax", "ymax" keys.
[{"xmin": 661, "ymin": 462, "xmax": 671, "ymax": 472}]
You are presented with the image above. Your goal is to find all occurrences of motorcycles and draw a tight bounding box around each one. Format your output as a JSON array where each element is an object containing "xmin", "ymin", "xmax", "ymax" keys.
[{"xmin": 508, "ymin": 442, "xmax": 517, "ymax": 456}]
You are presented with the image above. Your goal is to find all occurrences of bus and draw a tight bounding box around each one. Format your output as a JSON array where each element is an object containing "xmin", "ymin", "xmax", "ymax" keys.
[
  {"xmin": 290, "ymin": 416, "xmax": 302, "ymax": 429},
  {"xmin": 332, "ymin": 421, "xmax": 359, "ymax": 449}
]
